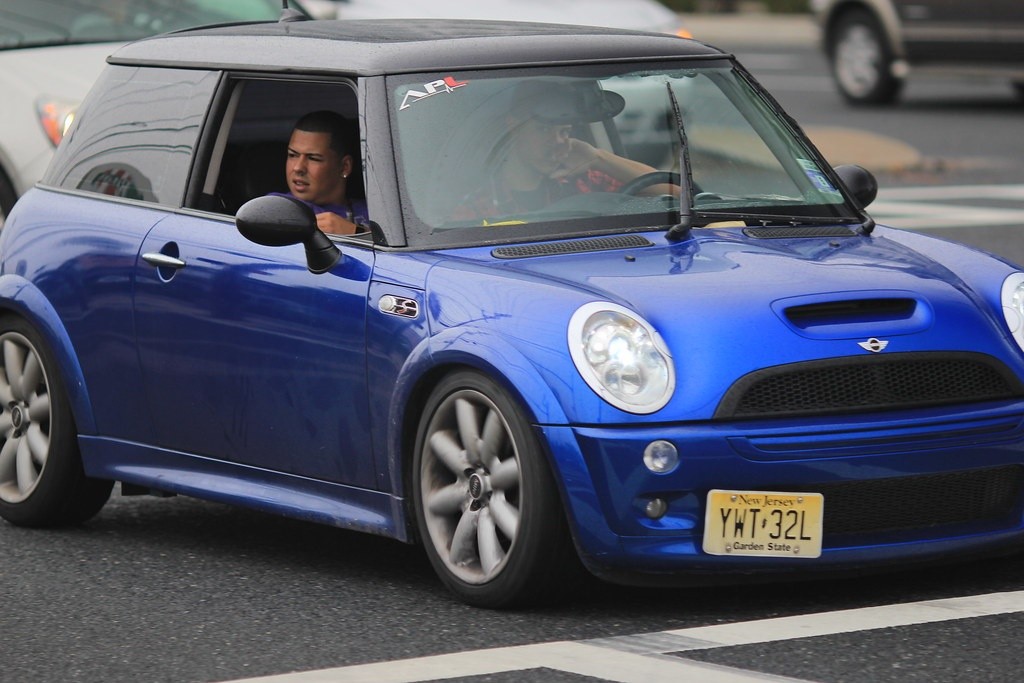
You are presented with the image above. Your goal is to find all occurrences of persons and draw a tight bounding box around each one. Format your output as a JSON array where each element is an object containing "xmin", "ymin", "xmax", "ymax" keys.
[
  {"xmin": 286, "ymin": 110, "xmax": 370, "ymax": 237},
  {"xmin": 456, "ymin": 81, "xmax": 679, "ymax": 219}
]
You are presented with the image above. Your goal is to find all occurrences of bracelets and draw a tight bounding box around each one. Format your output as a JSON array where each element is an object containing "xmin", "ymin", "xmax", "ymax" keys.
[{"xmin": 355, "ymin": 223, "xmax": 365, "ymax": 234}]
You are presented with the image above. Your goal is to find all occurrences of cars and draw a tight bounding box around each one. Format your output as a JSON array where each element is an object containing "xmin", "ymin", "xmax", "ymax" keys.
[
  {"xmin": 808, "ymin": 0, "xmax": 1024, "ymax": 112},
  {"xmin": 0, "ymin": 0, "xmax": 341, "ymax": 227},
  {"xmin": 0, "ymin": 11, "xmax": 1024, "ymax": 610},
  {"xmin": 204, "ymin": 2, "xmax": 692, "ymax": 165}
]
